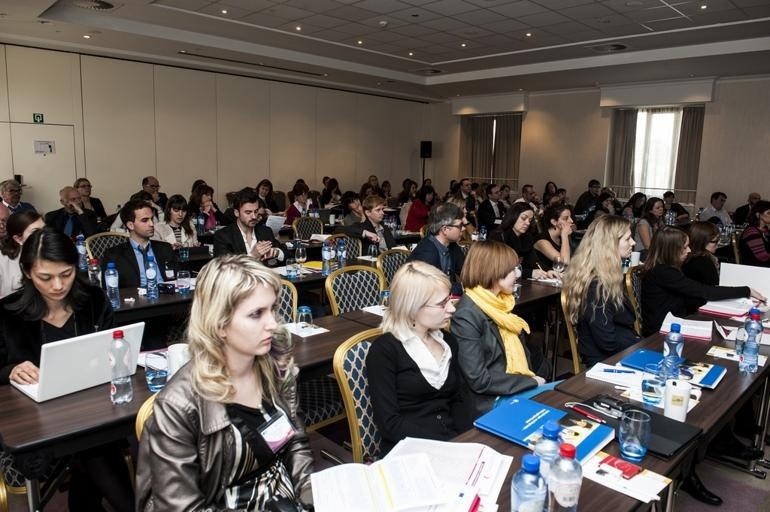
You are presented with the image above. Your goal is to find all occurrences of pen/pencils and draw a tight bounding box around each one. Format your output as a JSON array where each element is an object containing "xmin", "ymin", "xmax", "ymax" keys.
[
  {"xmin": 565, "ymin": 403, "xmax": 607, "ymax": 425},
  {"xmin": 600, "ymin": 369, "xmax": 634, "ymax": 373}
]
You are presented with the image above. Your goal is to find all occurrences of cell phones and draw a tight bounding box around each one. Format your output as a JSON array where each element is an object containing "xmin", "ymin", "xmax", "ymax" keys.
[{"xmin": 593, "ymin": 393, "xmax": 629, "ymax": 418}]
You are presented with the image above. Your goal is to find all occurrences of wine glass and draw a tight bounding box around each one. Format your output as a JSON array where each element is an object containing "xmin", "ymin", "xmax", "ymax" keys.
[
  {"xmin": 552, "ymin": 256, "xmax": 566, "ymax": 289},
  {"xmin": 295, "ymin": 247, "xmax": 308, "ymax": 278}
]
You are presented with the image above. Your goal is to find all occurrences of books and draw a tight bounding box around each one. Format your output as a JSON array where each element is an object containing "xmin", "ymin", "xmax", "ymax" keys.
[
  {"xmin": 583, "ymin": 345, "xmax": 729, "ymax": 397},
  {"xmin": 473, "ymin": 396, "xmax": 618, "ymax": 474},
  {"xmin": 698, "ymin": 290, "xmax": 755, "ymax": 320},
  {"xmin": 309, "ymin": 433, "xmax": 514, "ymax": 511},
  {"xmin": 659, "ymin": 309, "xmax": 716, "ymax": 343}
]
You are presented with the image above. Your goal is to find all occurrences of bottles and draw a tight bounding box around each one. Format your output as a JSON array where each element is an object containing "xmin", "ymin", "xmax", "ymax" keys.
[
  {"xmin": 191, "ymin": 212, "xmax": 205, "ymax": 235},
  {"xmin": 510, "ymin": 420, "xmax": 582, "ymax": 512},
  {"xmin": 736, "ymin": 308, "xmax": 764, "ymax": 373},
  {"xmin": 76, "ymin": 235, "xmax": 87, "ymax": 271},
  {"xmin": 719, "ymin": 223, "xmax": 736, "ymax": 245},
  {"xmin": 471, "ymin": 226, "xmax": 487, "ymax": 243},
  {"xmin": 109, "ymin": 330, "xmax": 133, "ymax": 404},
  {"xmin": 144, "ymin": 255, "xmax": 160, "ymax": 299},
  {"xmin": 582, "ymin": 210, "xmax": 588, "ymax": 221},
  {"xmin": 321, "ymin": 239, "xmax": 347, "ymax": 277},
  {"xmin": 513, "ymin": 263, "xmax": 523, "ymax": 298},
  {"xmin": 662, "ymin": 322, "xmax": 685, "ymax": 381},
  {"xmin": 665, "ymin": 210, "xmax": 677, "ymax": 226},
  {"xmin": 105, "ymin": 263, "xmax": 121, "ymax": 310},
  {"xmin": 382, "ymin": 214, "xmax": 401, "ymax": 238},
  {"xmin": 88, "ymin": 258, "xmax": 102, "ymax": 288},
  {"xmin": 301, "ymin": 208, "xmax": 306, "ymax": 217}
]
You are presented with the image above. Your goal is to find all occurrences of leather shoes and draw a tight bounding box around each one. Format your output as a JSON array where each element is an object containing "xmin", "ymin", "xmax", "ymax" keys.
[
  {"xmin": 682, "ymin": 474, "xmax": 722, "ymax": 505},
  {"xmin": 708, "ymin": 437, "xmax": 764, "ymax": 460}
]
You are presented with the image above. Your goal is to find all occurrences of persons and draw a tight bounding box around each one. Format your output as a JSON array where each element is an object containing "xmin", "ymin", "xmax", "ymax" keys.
[
  {"xmin": 576, "ymin": 215, "xmax": 764, "ymax": 460},
  {"xmin": 452, "ymin": 239, "xmax": 725, "ymax": 507},
  {"xmin": 0, "ymin": 226, "xmax": 139, "ymax": 511},
  {"xmin": 641, "ymin": 224, "xmax": 769, "ymax": 443},
  {"xmin": 135, "ymin": 254, "xmax": 316, "ymax": 512},
  {"xmin": 0, "ymin": 170, "xmax": 770, "ymax": 297},
  {"xmin": 367, "ymin": 261, "xmax": 479, "ymax": 455}
]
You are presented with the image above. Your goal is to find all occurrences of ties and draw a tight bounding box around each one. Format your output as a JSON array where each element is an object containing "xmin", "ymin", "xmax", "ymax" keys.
[
  {"xmin": 64, "ymin": 214, "xmax": 72, "ymax": 238},
  {"xmin": 138, "ymin": 244, "xmax": 149, "ymax": 273}
]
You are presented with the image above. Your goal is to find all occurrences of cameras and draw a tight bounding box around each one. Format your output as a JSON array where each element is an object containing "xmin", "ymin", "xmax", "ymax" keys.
[{"xmin": 158, "ymin": 283, "xmax": 175, "ymax": 295}]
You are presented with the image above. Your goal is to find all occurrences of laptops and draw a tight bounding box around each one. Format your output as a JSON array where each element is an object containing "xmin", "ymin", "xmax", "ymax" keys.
[{"xmin": 9, "ymin": 320, "xmax": 145, "ymax": 404}]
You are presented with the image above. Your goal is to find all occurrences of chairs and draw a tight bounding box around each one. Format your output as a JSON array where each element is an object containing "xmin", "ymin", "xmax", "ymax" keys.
[
  {"xmin": 84, "ymin": 233, "xmax": 130, "ymax": 264},
  {"xmin": 625, "ymin": 264, "xmax": 643, "ymax": 336},
  {"xmin": 560, "ymin": 288, "xmax": 585, "ymax": 375},
  {"xmin": 376, "ymin": 249, "xmax": 411, "ymax": 287},
  {"xmin": 325, "ymin": 265, "xmax": 384, "ymax": 316},
  {"xmin": 730, "ymin": 232, "xmax": 740, "ymax": 265},
  {"xmin": 292, "ymin": 216, "xmax": 324, "ymax": 239},
  {"xmin": 0, "ymin": 177, "xmax": 769, "ymax": 511},
  {"xmin": 326, "ymin": 233, "xmax": 363, "ymax": 259}
]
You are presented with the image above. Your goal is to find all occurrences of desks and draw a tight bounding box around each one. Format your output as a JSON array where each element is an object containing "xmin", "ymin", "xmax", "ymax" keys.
[
  {"xmin": 106, "ymin": 256, "xmax": 376, "ymax": 324},
  {"xmin": 0, "ymin": 280, "xmax": 560, "ymax": 512},
  {"xmin": 448, "ymin": 311, "xmax": 769, "ymax": 512}
]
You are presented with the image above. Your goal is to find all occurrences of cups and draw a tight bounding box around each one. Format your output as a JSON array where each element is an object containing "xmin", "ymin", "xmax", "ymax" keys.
[
  {"xmin": 296, "ymin": 305, "xmax": 313, "ymax": 330},
  {"xmin": 175, "ymin": 271, "xmax": 192, "ymax": 295},
  {"xmin": 661, "ymin": 378, "xmax": 701, "ymax": 421},
  {"xmin": 630, "ymin": 251, "xmax": 641, "ymax": 266},
  {"xmin": 410, "ymin": 244, "xmax": 418, "ymax": 252},
  {"xmin": 617, "ymin": 409, "xmax": 651, "ymax": 463},
  {"xmin": 329, "ymin": 214, "xmax": 336, "ymax": 224},
  {"xmin": 368, "ymin": 244, "xmax": 378, "ymax": 259},
  {"xmin": 145, "ymin": 351, "xmax": 168, "ymax": 393},
  {"xmin": 284, "ymin": 258, "xmax": 299, "ymax": 279},
  {"xmin": 309, "ymin": 212, "xmax": 320, "ymax": 218},
  {"xmin": 379, "ymin": 290, "xmax": 390, "ymax": 310},
  {"xmin": 178, "ymin": 242, "xmax": 190, "ymax": 259},
  {"xmin": 642, "ymin": 362, "xmax": 667, "ymax": 404},
  {"xmin": 169, "ymin": 342, "xmax": 191, "ymax": 377}
]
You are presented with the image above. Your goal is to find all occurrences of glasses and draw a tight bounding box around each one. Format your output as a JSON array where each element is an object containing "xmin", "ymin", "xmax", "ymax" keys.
[
  {"xmin": 424, "ymin": 293, "xmax": 451, "ymax": 308},
  {"xmin": 145, "ymin": 184, "xmax": 160, "ymax": 189},
  {"xmin": 710, "ymin": 239, "xmax": 720, "ymax": 244},
  {"xmin": 441, "ymin": 222, "xmax": 462, "ymax": 230}
]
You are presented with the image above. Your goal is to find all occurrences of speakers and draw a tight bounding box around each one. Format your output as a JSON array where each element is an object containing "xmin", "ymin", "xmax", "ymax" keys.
[{"xmin": 421, "ymin": 141, "xmax": 432, "ymax": 158}]
